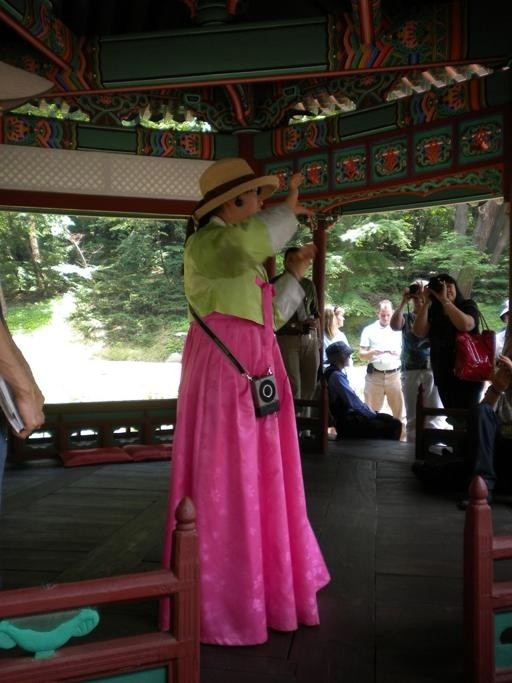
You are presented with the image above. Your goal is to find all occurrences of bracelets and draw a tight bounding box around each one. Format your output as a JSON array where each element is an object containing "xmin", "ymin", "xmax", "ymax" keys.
[
  {"xmin": 441, "ymin": 297, "xmax": 451, "ymax": 309},
  {"xmin": 424, "ymin": 299, "xmax": 433, "ymax": 309},
  {"xmin": 487, "ymin": 385, "xmax": 506, "ymax": 398}
]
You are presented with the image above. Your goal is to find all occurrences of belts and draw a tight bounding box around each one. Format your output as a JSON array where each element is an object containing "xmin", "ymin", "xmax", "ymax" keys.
[{"xmin": 372, "ymin": 367, "xmax": 402, "ymax": 374}]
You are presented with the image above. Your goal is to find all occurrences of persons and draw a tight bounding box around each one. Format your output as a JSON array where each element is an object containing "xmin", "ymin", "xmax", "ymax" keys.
[
  {"xmin": 318, "ymin": 341, "xmax": 403, "ymax": 444},
  {"xmin": 321, "ymin": 304, "xmax": 353, "ymax": 379},
  {"xmin": 457, "ymin": 346, "xmax": 512, "ymax": 512},
  {"xmin": 358, "ymin": 298, "xmax": 407, "ymax": 444},
  {"xmin": 483, "ymin": 297, "xmax": 511, "ymax": 393},
  {"xmin": 411, "ymin": 274, "xmax": 483, "ymax": 462},
  {"xmin": 1, "ymin": 307, "xmax": 46, "ymax": 485},
  {"xmin": 269, "ymin": 247, "xmax": 324, "ymax": 438},
  {"xmin": 158, "ymin": 160, "xmax": 333, "ymax": 646},
  {"xmin": 390, "ymin": 278, "xmax": 447, "ymax": 445}
]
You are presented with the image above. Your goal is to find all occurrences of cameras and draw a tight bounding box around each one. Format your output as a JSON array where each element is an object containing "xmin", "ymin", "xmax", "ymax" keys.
[
  {"xmin": 408, "ymin": 284, "xmax": 420, "ymax": 295},
  {"xmin": 428, "ymin": 277, "xmax": 443, "ymax": 293}
]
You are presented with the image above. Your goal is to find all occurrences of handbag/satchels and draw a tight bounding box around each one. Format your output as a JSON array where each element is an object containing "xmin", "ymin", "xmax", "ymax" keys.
[
  {"xmin": 456, "ymin": 330, "xmax": 496, "ymax": 381},
  {"xmin": 252, "ymin": 374, "xmax": 281, "ymax": 417}
]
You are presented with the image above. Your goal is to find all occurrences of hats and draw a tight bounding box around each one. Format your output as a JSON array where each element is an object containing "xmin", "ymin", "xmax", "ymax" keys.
[
  {"xmin": 499, "ymin": 300, "xmax": 510, "ymax": 318},
  {"xmin": 320, "ymin": 341, "xmax": 355, "ymax": 364},
  {"xmin": 193, "ymin": 156, "xmax": 280, "ymax": 219}
]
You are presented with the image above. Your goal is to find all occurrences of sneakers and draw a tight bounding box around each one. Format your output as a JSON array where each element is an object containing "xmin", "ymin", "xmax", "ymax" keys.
[{"xmin": 459, "ymin": 476, "xmax": 494, "ymax": 508}]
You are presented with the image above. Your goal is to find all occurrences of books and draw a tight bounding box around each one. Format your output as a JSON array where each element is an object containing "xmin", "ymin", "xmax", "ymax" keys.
[{"xmin": 1, "ymin": 377, "xmax": 24, "ymax": 434}]
